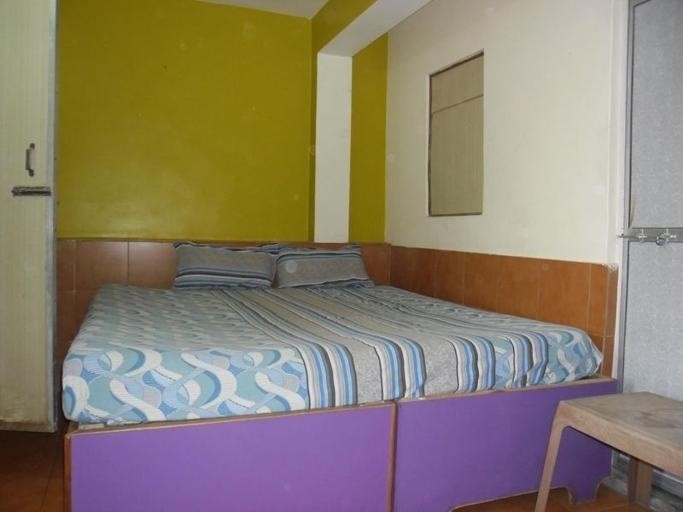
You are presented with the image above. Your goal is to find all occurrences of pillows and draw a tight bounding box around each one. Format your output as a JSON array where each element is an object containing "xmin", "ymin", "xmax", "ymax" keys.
[{"xmin": 170, "ymin": 238, "xmax": 376, "ymax": 290}]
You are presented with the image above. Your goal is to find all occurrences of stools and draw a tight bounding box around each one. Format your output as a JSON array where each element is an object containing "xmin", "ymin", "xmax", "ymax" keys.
[{"xmin": 533, "ymin": 390, "xmax": 683, "ymax": 512}]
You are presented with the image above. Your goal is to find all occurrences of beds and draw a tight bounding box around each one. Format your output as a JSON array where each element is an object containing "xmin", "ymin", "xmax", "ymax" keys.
[{"xmin": 63, "ymin": 283, "xmax": 616, "ymax": 512}]
